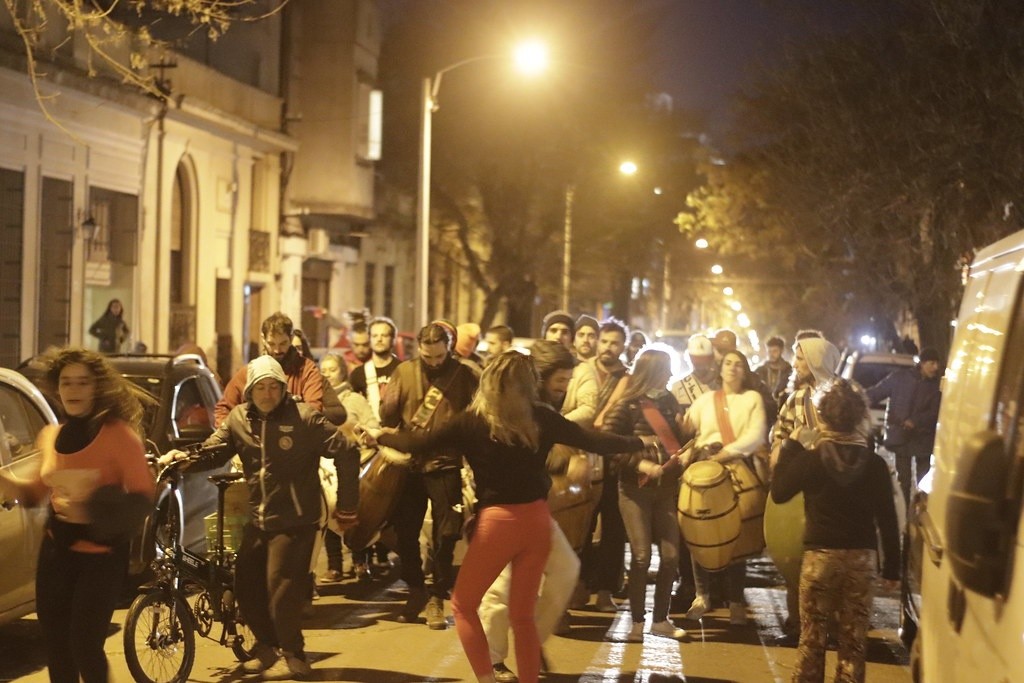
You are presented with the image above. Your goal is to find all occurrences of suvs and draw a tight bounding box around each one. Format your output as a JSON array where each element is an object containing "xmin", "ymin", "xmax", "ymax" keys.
[
  {"xmin": 840, "ymin": 351, "xmax": 920, "ymax": 438},
  {"xmin": 14, "ymin": 348, "xmax": 237, "ymax": 571}
]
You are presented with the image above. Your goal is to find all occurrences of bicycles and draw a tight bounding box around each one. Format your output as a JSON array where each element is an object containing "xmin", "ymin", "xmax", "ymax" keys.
[{"xmin": 123, "ymin": 443, "xmax": 259, "ymax": 683}]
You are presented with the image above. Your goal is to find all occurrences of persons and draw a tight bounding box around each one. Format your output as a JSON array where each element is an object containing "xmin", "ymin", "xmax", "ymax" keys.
[
  {"xmin": 90, "ymin": 298, "xmax": 129, "ymax": 353},
  {"xmin": 0, "ymin": 344, "xmax": 157, "ymax": 683},
  {"xmin": 159, "ymin": 310, "xmax": 942, "ymax": 683}
]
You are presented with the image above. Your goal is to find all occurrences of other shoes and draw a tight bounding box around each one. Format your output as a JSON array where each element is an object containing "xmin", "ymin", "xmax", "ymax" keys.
[
  {"xmin": 567, "ymin": 588, "xmax": 618, "ymax": 612},
  {"xmin": 424, "ymin": 572, "xmax": 434, "ymax": 584},
  {"xmin": 366, "ymin": 561, "xmax": 390, "ymax": 576},
  {"xmin": 260, "ymin": 652, "xmax": 312, "ymax": 680},
  {"xmin": 312, "ymin": 581, "xmax": 319, "ymax": 599},
  {"xmin": 320, "ymin": 567, "xmax": 344, "ymax": 582},
  {"xmin": 241, "ymin": 645, "xmax": 277, "ymax": 670},
  {"xmin": 355, "ymin": 566, "xmax": 369, "ymax": 586}
]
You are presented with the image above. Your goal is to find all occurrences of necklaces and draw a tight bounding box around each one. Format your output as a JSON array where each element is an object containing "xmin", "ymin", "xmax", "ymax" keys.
[{"xmin": 721, "ymin": 391, "xmax": 736, "ymax": 413}]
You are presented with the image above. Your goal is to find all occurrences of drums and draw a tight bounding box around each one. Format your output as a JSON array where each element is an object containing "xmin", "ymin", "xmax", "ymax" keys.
[
  {"xmin": 676, "ymin": 460, "xmax": 766, "ymax": 575},
  {"xmin": 541, "ymin": 448, "xmax": 605, "ymax": 557},
  {"xmin": 342, "ymin": 443, "xmax": 413, "ymax": 551}
]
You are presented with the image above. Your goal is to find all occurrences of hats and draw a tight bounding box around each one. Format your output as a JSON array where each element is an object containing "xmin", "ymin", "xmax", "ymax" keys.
[
  {"xmin": 716, "ymin": 330, "xmax": 736, "ymax": 350},
  {"xmin": 919, "ymin": 347, "xmax": 943, "ymax": 361},
  {"xmin": 629, "ymin": 332, "xmax": 646, "ymax": 346},
  {"xmin": 688, "ymin": 334, "xmax": 713, "ymax": 357},
  {"xmin": 243, "ymin": 355, "xmax": 288, "ymax": 400},
  {"xmin": 455, "ymin": 323, "xmax": 481, "ymax": 358},
  {"xmin": 575, "ymin": 313, "xmax": 600, "ymax": 339},
  {"xmin": 541, "ymin": 311, "xmax": 576, "ymax": 343},
  {"xmin": 431, "ymin": 319, "xmax": 458, "ymax": 353}
]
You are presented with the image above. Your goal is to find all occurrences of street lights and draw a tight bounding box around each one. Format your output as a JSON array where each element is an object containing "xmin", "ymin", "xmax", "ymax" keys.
[
  {"xmin": 414, "ymin": 42, "xmax": 551, "ymax": 359},
  {"xmin": 561, "ymin": 158, "xmax": 638, "ymax": 315}
]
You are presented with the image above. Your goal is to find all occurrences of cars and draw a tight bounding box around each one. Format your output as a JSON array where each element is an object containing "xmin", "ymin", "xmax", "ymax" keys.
[{"xmin": 0, "ymin": 368, "xmax": 58, "ymax": 627}]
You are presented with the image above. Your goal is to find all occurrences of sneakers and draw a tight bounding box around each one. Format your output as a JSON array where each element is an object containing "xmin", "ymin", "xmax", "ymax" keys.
[
  {"xmin": 426, "ymin": 594, "xmax": 449, "ymax": 630},
  {"xmin": 492, "ymin": 662, "xmax": 518, "ymax": 683},
  {"xmin": 730, "ymin": 602, "xmax": 748, "ymax": 626},
  {"xmin": 651, "ymin": 619, "xmax": 686, "ymax": 638},
  {"xmin": 396, "ymin": 584, "xmax": 431, "ymax": 623},
  {"xmin": 627, "ymin": 622, "xmax": 645, "ymax": 642},
  {"xmin": 685, "ymin": 593, "xmax": 712, "ymax": 620},
  {"xmin": 538, "ymin": 645, "xmax": 549, "ymax": 679}
]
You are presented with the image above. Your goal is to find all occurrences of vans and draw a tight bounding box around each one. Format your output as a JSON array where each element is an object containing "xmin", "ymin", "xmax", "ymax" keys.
[{"xmin": 915, "ymin": 228, "xmax": 1024, "ymax": 683}]
